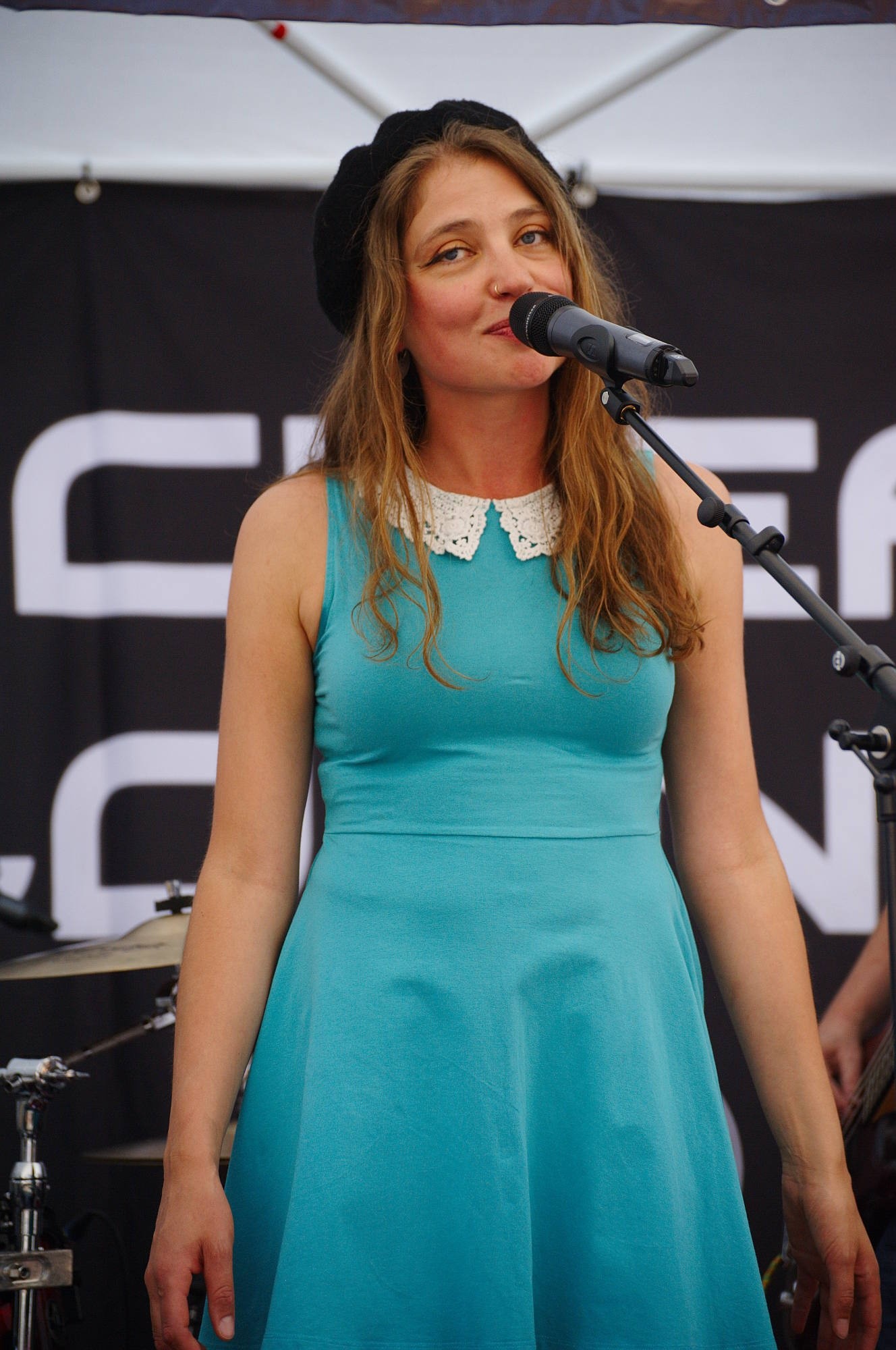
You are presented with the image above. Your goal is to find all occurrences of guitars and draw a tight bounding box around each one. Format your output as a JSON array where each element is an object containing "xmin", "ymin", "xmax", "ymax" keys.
[{"xmin": 789, "ymin": 1010, "xmax": 896, "ymax": 1335}]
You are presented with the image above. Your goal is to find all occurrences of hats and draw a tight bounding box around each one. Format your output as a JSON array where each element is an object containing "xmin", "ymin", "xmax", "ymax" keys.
[{"xmin": 313, "ymin": 98, "xmax": 571, "ymax": 340}]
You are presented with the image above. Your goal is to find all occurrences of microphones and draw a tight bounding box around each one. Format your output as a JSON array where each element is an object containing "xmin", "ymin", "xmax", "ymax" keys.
[{"xmin": 508, "ymin": 292, "xmax": 699, "ymax": 388}]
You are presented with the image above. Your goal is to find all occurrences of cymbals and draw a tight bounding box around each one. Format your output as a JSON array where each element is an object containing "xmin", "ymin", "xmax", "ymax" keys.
[
  {"xmin": 0, "ymin": 911, "xmax": 191, "ymax": 982},
  {"xmin": 79, "ymin": 1120, "xmax": 238, "ymax": 1166}
]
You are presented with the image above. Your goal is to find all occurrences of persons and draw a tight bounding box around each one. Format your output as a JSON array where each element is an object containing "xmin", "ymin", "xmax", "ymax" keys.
[
  {"xmin": 818, "ymin": 903, "xmax": 888, "ymax": 1121},
  {"xmin": 145, "ymin": 100, "xmax": 881, "ymax": 1350}
]
